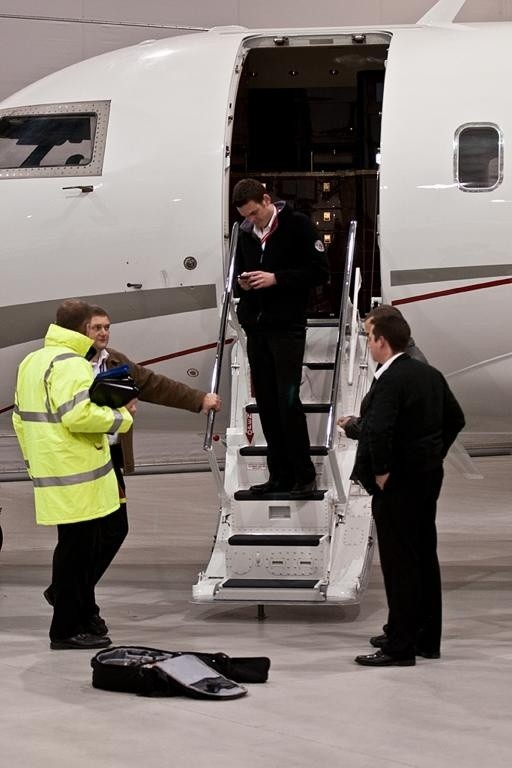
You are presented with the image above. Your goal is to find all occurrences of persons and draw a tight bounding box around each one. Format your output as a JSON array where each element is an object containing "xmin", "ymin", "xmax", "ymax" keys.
[
  {"xmin": 13, "ymin": 300, "xmax": 135, "ymax": 648},
  {"xmin": 85, "ymin": 306, "xmax": 223, "ymax": 508},
  {"xmin": 337, "ymin": 305, "xmax": 466, "ymax": 665},
  {"xmin": 232, "ymin": 178, "xmax": 324, "ymax": 494}
]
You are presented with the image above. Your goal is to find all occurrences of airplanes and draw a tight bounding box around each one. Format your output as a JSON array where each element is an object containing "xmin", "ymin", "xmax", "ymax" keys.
[{"xmin": 0, "ymin": 0, "xmax": 512, "ymax": 621}]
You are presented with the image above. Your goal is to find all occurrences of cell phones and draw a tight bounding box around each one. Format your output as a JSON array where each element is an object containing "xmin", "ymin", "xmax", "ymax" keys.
[{"xmin": 237, "ymin": 274, "xmax": 251, "ymax": 280}]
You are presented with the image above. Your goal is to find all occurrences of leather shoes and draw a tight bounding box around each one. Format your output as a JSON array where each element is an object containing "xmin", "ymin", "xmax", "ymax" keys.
[
  {"xmin": 370, "ymin": 624, "xmax": 442, "ymax": 659},
  {"xmin": 355, "ymin": 651, "xmax": 417, "ymax": 667},
  {"xmin": 250, "ymin": 479, "xmax": 318, "ymax": 494},
  {"xmin": 42, "ymin": 584, "xmax": 112, "ymax": 648}
]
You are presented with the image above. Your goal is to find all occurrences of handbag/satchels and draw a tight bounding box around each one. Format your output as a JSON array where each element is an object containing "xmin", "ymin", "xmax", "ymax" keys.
[{"xmin": 88, "ymin": 642, "xmax": 272, "ymax": 701}]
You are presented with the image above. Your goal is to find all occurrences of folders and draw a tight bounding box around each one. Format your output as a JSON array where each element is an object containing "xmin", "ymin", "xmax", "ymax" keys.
[{"xmin": 89, "ymin": 365, "xmax": 141, "ymax": 409}]
